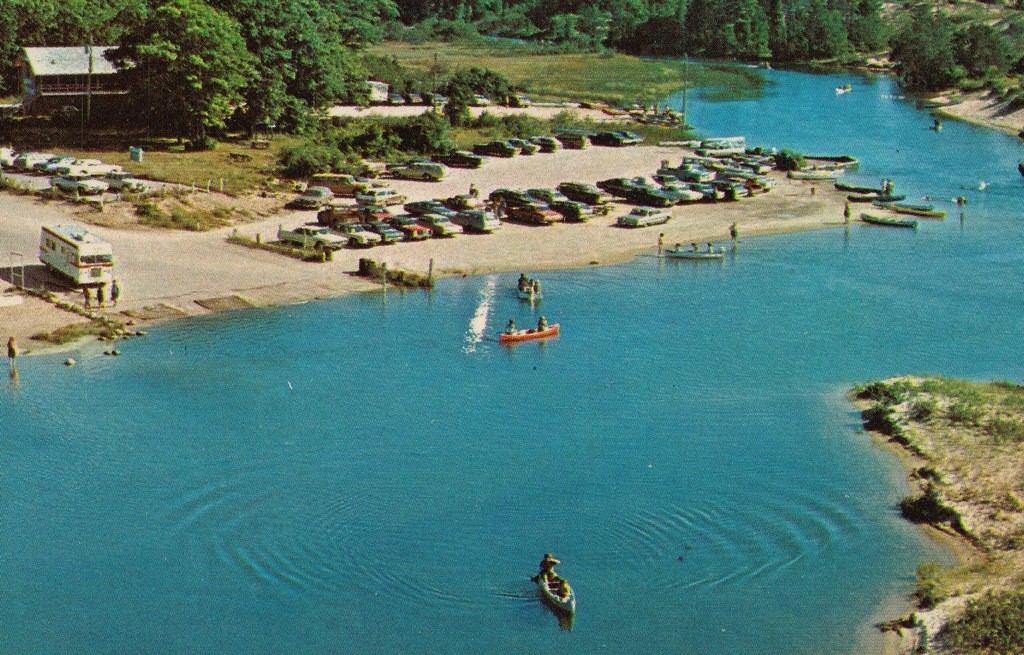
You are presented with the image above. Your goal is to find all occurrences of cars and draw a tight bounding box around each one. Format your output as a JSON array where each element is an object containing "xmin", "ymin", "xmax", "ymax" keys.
[
  {"xmin": 300, "ymin": 187, "xmax": 334, "ymax": 211},
  {"xmin": 0, "ymin": 148, "xmax": 147, "ymax": 196},
  {"xmin": 596, "ymin": 155, "xmax": 775, "ymax": 207},
  {"xmin": 386, "ymin": 130, "xmax": 643, "ymax": 181},
  {"xmin": 489, "ymin": 182, "xmax": 612, "ymax": 227},
  {"xmin": 518, "ymin": 96, "xmax": 531, "ymax": 106},
  {"xmin": 466, "ymin": 93, "xmax": 490, "ymax": 107},
  {"xmin": 356, "ymin": 189, "xmax": 406, "ymax": 208},
  {"xmin": 276, "ymin": 194, "xmax": 499, "ymax": 252},
  {"xmin": 617, "ymin": 206, "xmax": 668, "ymax": 228}
]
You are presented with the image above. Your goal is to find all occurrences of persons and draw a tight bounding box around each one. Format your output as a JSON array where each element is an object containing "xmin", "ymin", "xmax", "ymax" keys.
[
  {"xmin": 729, "ymin": 222, "xmax": 738, "ymax": 242},
  {"xmin": 557, "ymin": 579, "xmax": 570, "ymax": 603},
  {"xmin": 690, "ymin": 242, "xmax": 699, "ymax": 253},
  {"xmin": 658, "ymin": 233, "xmax": 664, "ymax": 254},
  {"xmin": 505, "ymin": 319, "xmax": 516, "ymax": 335},
  {"xmin": 707, "ymin": 241, "xmax": 715, "ymax": 253},
  {"xmin": 110, "ymin": 279, "xmax": 119, "ymax": 308},
  {"xmin": 97, "ymin": 284, "xmax": 105, "ymax": 310},
  {"xmin": 641, "ymin": 103, "xmax": 670, "ymax": 119},
  {"xmin": 518, "ymin": 273, "xmax": 541, "ymax": 295},
  {"xmin": 880, "ymin": 178, "xmax": 894, "ymax": 196},
  {"xmin": 843, "ymin": 202, "xmax": 851, "ymax": 224},
  {"xmin": 79, "ymin": 287, "xmax": 92, "ymax": 310},
  {"xmin": 539, "ymin": 553, "xmax": 560, "ymax": 583},
  {"xmin": 537, "ymin": 316, "xmax": 547, "ymax": 331},
  {"xmin": 675, "ymin": 243, "xmax": 682, "ymax": 253},
  {"xmin": 469, "ymin": 183, "xmax": 506, "ymax": 217},
  {"xmin": 7, "ymin": 337, "xmax": 18, "ymax": 366}
]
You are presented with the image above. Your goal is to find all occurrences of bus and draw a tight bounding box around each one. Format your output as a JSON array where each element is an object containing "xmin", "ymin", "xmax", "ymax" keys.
[{"xmin": 38, "ymin": 224, "xmax": 114, "ymax": 286}]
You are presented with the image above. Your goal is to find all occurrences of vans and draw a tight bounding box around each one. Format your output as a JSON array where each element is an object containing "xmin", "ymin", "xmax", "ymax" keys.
[
  {"xmin": 694, "ymin": 136, "xmax": 747, "ymax": 158},
  {"xmin": 307, "ymin": 174, "xmax": 369, "ymax": 198}
]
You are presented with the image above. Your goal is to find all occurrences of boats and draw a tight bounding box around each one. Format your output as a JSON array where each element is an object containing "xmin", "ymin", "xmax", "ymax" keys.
[
  {"xmin": 665, "ymin": 246, "xmax": 727, "ymax": 259},
  {"xmin": 835, "ymin": 182, "xmax": 947, "ymax": 219},
  {"xmin": 499, "ymin": 323, "xmax": 559, "ymax": 346},
  {"xmin": 516, "ymin": 285, "xmax": 544, "ymax": 300},
  {"xmin": 787, "ymin": 170, "xmax": 845, "ymax": 180},
  {"xmin": 861, "ymin": 212, "xmax": 920, "ymax": 227},
  {"xmin": 538, "ymin": 572, "xmax": 575, "ymax": 611}
]
